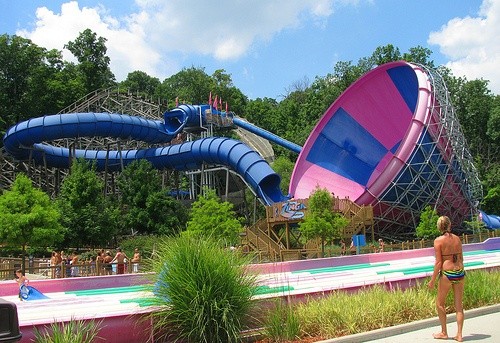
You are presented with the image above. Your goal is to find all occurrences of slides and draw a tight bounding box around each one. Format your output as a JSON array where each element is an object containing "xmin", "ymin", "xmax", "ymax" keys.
[
  {"xmin": 478, "ymin": 211, "xmax": 500, "ymax": 230},
  {"xmin": 233, "ymin": 116, "xmax": 303, "ymax": 154},
  {"xmin": 3, "ymin": 105, "xmax": 292, "ymax": 208}
]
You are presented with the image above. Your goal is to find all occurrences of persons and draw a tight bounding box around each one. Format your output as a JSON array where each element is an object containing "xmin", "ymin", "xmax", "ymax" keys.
[
  {"xmin": 50, "ymin": 248, "xmax": 140, "ymax": 277},
  {"xmin": 15, "ymin": 269, "xmax": 29, "ymax": 299},
  {"xmin": 291, "ymin": 196, "xmax": 295, "ymax": 201},
  {"xmin": 428, "ymin": 215, "xmax": 466, "ymax": 342},
  {"xmin": 175, "ymin": 133, "xmax": 183, "ymax": 143},
  {"xmin": 340, "ymin": 238, "xmax": 356, "ymax": 256},
  {"xmin": 331, "ymin": 193, "xmax": 335, "ymax": 199},
  {"xmin": 222, "ymin": 243, "xmax": 249, "ymax": 255},
  {"xmin": 370, "ymin": 238, "xmax": 385, "ymax": 253}
]
